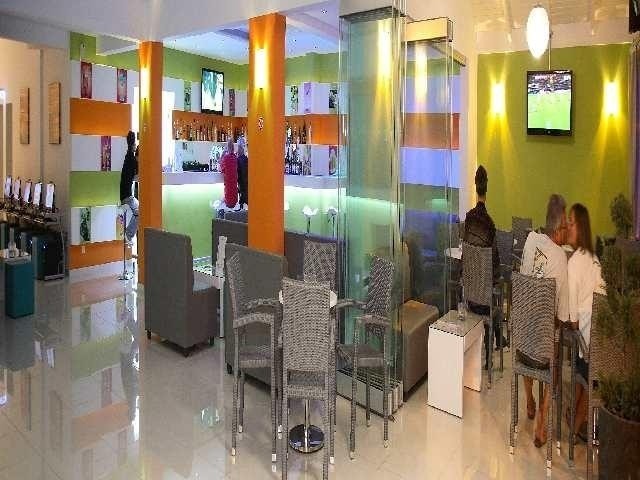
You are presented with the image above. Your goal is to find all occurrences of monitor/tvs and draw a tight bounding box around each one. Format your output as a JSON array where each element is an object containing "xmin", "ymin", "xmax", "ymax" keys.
[
  {"xmin": 201, "ymin": 67, "xmax": 224, "ymax": 115},
  {"xmin": 526, "ymin": 70, "xmax": 573, "ymax": 135}
]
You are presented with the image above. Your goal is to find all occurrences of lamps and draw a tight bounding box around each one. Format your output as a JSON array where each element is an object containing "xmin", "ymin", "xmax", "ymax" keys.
[{"xmin": 523, "ymin": 0, "xmax": 551, "ymax": 58}]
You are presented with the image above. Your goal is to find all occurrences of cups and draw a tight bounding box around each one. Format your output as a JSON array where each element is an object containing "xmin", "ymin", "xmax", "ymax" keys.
[
  {"xmin": 455, "ymin": 284, "xmax": 470, "ymax": 320},
  {"xmin": 6, "ymin": 241, "xmax": 17, "ymax": 258}
]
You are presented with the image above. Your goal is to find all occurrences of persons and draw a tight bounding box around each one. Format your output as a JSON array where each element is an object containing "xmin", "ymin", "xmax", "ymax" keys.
[
  {"xmin": 120, "ymin": 131, "xmax": 140, "ymax": 245},
  {"xmin": 211, "ymin": 137, "xmax": 241, "ymax": 211},
  {"xmin": 133, "ymin": 132, "xmax": 139, "ymax": 237},
  {"xmin": 565, "ymin": 203, "xmax": 608, "ymax": 446},
  {"xmin": 460, "ymin": 165, "xmax": 507, "ymax": 369},
  {"xmin": 235, "ymin": 138, "xmax": 248, "ymax": 211},
  {"xmin": 518, "ymin": 193, "xmax": 567, "ymax": 447}
]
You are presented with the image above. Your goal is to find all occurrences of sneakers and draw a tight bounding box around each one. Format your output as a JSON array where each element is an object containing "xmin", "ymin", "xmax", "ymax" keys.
[{"xmin": 120, "ymin": 231, "xmax": 135, "ymax": 246}]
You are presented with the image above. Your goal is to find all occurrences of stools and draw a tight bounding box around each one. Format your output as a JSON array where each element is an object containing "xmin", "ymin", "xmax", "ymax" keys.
[
  {"xmin": 114, "ymin": 200, "xmax": 136, "ymax": 281},
  {"xmin": 0, "ymin": 217, "xmax": 65, "ymax": 319}
]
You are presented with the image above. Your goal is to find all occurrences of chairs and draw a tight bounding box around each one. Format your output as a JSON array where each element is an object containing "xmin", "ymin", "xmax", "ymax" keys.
[
  {"xmin": 142, "ymin": 227, "xmax": 220, "ymax": 357},
  {"xmin": 508, "ymin": 269, "xmax": 565, "ymax": 472},
  {"xmin": 587, "ymin": 291, "xmax": 640, "ymax": 480},
  {"xmin": 209, "ymin": 203, "xmax": 393, "ymax": 480},
  {"xmin": 460, "ymin": 216, "xmax": 531, "ymax": 390}
]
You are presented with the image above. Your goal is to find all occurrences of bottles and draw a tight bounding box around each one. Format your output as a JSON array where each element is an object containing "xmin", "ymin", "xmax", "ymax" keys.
[
  {"xmin": 328, "ymin": 149, "xmax": 337, "ymax": 174},
  {"xmin": 174, "ymin": 118, "xmax": 249, "ymax": 142},
  {"xmin": 284, "ymin": 119, "xmax": 313, "ymax": 177}
]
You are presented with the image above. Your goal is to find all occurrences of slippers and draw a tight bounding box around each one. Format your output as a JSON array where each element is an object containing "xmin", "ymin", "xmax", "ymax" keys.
[{"xmin": 527, "ymin": 402, "xmax": 547, "ymax": 448}]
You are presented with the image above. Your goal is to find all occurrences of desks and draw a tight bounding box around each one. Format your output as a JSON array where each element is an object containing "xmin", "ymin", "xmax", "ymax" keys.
[{"xmin": 1, "ymin": 204, "xmax": 68, "ymax": 281}]
